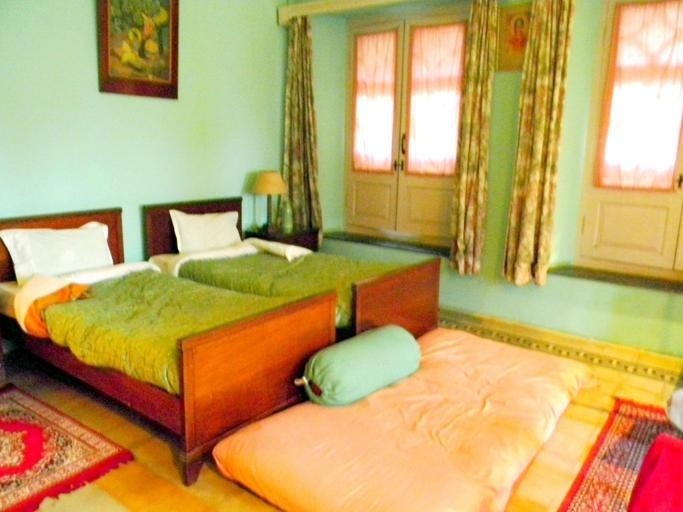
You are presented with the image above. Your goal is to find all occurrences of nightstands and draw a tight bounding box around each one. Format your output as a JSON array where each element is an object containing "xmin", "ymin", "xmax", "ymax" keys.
[{"xmin": 244, "ymin": 225, "xmax": 318, "ymax": 251}]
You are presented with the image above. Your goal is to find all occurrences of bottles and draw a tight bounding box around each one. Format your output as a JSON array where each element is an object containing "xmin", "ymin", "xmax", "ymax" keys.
[{"xmin": 280, "ymin": 200, "xmax": 293, "ymax": 235}]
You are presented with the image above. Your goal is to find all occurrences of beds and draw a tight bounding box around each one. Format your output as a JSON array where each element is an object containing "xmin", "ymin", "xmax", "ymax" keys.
[
  {"xmin": 0, "ymin": 207, "xmax": 337, "ymax": 486},
  {"xmin": 140, "ymin": 196, "xmax": 441, "ymax": 341}
]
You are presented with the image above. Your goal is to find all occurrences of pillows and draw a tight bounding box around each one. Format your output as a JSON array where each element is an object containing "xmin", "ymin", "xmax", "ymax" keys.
[
  {"xmin": 293, "ymin": 323, "xmax": 420, "ymax": 407},
  {"xmin": 169, "ymin": 209, "xmax": 242, "ymax": 255},
  {"xmin": 0, "ymin": 220, "xmax": 113, "ymax": 286}
]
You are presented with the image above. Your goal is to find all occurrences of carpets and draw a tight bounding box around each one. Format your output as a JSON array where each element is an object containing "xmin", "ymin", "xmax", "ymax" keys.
[
  {"xmin": 558, "ymin": 397, "xmax": 683, "ymax": 512},
  {"xmin": 0, "ymin": 381, "xmax": 136, "ymax": 512}
]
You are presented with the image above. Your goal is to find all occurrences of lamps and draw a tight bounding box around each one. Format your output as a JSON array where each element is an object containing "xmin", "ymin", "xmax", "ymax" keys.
[{"xmin": 249, "ymin": 171, "xmax": 289, "ymax": 239}]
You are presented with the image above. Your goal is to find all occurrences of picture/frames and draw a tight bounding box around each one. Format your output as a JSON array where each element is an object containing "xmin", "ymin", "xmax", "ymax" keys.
[
  {"xmin": 97, "ymin": 0, "xmax": 178, "ymax": 99},
  {"xmin": 495, "ymin": 7, "xmax": 530, "ymax": 72}
]
